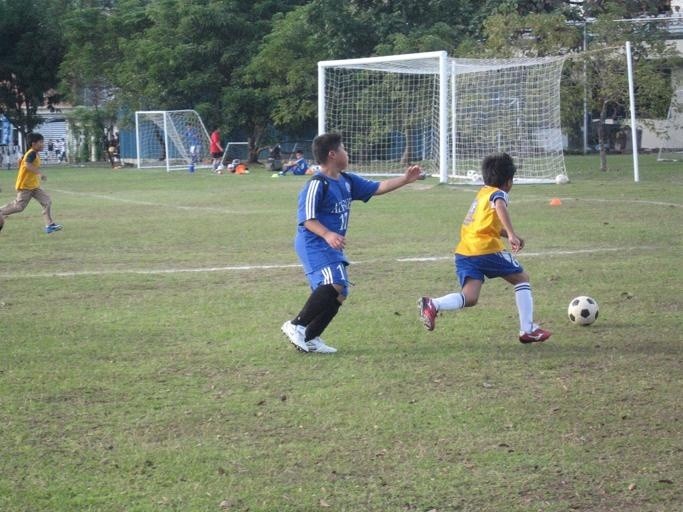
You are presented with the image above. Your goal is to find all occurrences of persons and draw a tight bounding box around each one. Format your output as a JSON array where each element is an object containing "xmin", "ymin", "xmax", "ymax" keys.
[
  {"xmin": 211, "ymin": 124, "xmax": 224, "ymax": 168},
  {"xmin": 271, "ymin": 150, "xmax": 307, "ymax": 178},
  {"xmin": 103, "ymin": 132, "xmax": 120, "ymax": 160},
  {"xmin": 182, "ymin": 122, "xmax": 202, "ymax": 162},
  {"xmin": 44, "ymin": 138, "xmax": 68, "ymax": 162},
  {"xmin": 0, "ymin": 133, "xmax": 63, "ymax": 233},
  {"xmin": 416, "ymin": 152, "xmax": 552, "ymax": 344},
  {"xmin": 281, "ymin": 133, "xmax": 423, "ymax": 354}
]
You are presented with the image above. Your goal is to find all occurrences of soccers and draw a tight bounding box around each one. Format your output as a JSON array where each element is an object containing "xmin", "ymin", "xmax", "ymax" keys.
[{"xmin": 568, "ymin": 296, "xmax": 599, "ymax": 326}]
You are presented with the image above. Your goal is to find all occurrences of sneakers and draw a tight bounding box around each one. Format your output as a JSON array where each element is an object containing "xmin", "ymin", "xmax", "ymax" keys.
[
  {"xmin": 519, "ymin": 329, "xmax": 552, "ymax": 343},
  {"xmin": 417, "ymin": 296, "xmax": 440, "ymax": 330},
  {"xmin": 281, "ymin": 320, "xmax": 309, "ymax": 353},
  {"xmin": 46, "ymin": 223, "xmax": 63, "ymax": 233},
  {"xmin": 305, "ymin": 336, "xmax": 338, "ymax": 353}
]
